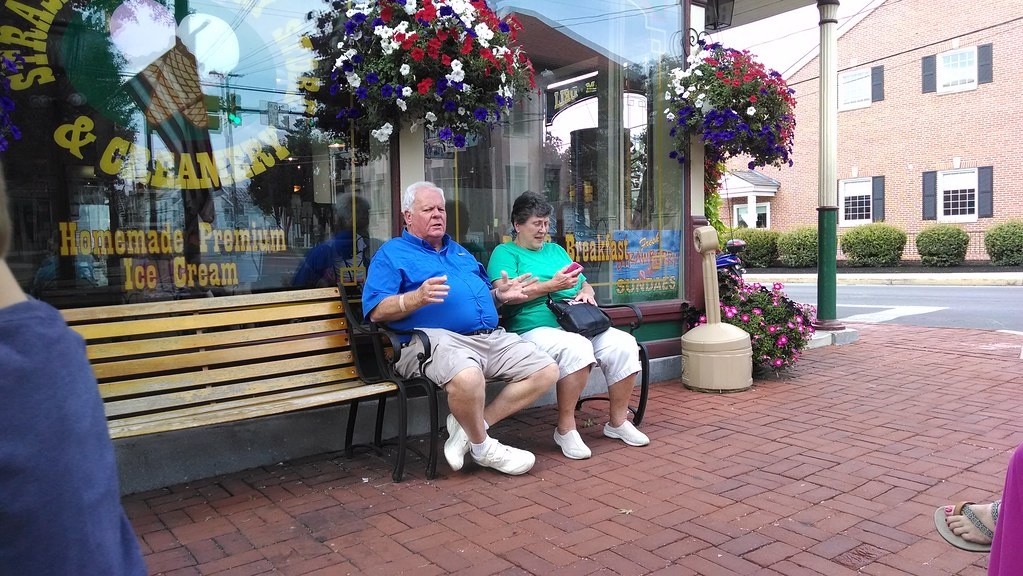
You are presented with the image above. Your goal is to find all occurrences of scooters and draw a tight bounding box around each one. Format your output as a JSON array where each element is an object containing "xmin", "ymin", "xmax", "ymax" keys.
[{"xmin": 714, "ymin": 238, "xmax": 748, "ymax": 293}]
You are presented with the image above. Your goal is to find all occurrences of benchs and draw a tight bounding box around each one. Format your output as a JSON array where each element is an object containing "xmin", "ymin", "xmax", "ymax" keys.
[
  {"xmin": 359, "ymin": 295, "xmax": 651, "ymax": 479},
  {"xmin": 57, "ymin": 281, "xmax": 409, "ymax": 482}
]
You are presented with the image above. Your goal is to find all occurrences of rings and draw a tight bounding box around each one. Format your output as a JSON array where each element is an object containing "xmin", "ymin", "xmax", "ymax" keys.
[{"xmin": 429, "ymin": 291, "xmax": 432, "ymax": 297}]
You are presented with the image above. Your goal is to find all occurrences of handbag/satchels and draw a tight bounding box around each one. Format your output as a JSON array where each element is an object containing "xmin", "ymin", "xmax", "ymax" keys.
[{"xmin": 547, "ymin": 299, "xmax": 614, "ymax": 338}]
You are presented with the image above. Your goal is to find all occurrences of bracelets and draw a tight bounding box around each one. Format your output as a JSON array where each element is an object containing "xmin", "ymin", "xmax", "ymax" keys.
[{"xmin": 494, "ymin": 290, "xmax": 510, "ymax": 304}]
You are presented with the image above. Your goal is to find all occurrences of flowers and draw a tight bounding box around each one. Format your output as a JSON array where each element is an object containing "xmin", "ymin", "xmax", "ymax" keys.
[
  {"xmin": 663, "ymin": 38, "xmax": 797, "ymax": 172},
  {"xmin": 688, "ymin": 275, "xmax": 817, "ymax": 378},
  {"xmin": 329, "ymin": 0, "xmax": 542, "ymax": 152}
]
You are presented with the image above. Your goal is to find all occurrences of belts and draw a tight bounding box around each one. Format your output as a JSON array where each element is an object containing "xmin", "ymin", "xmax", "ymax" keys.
[{"xmin": 462, "ymin": 329, "xmax": 495, "ymax": 336}]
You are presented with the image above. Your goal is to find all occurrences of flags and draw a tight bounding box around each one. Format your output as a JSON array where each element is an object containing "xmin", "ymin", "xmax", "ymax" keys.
[{"xmin": 123, "ymin": 36, "xmax": 222, "ymax": 225}]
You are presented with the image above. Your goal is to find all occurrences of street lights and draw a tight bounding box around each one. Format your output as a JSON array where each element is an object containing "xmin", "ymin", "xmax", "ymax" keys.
[{"xmin": 231, "ymin": 94, "xmax": 243, "ymax": 128}]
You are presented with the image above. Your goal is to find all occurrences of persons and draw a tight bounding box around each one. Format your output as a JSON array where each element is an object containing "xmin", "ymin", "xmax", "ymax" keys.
[
  {"xmin": 445, "ymin": 200, "xmax": 491, "ymax": 271},
  {"xmin": 291, "ymin": 193, "xmax": 382, "ymax": 290},
  {"xmin": 362, "ymin": 181, "xmax": 558, "ymax": 475},
  {"xmin": 0, "ymin": 165, "xmax": 149, "ymax": 576},
  {"xmin": 934, "ymin": 500, "xmax": 1002, "ymax": 553},
  {"xmin": 487, "ymin": 191, "xmax": 649, "ymax": 460}
]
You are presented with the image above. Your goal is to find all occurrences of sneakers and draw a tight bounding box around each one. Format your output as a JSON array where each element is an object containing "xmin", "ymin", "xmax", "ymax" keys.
[
  {"xmin": 444, "ymin": 413, "xmax": 471, "ymax": 472},
  {"xmin": 603, "ymin": 420, "xmax": 650, "ymax": 446},
  {"xmin": 470, "ymin": 438, "xmax": 535, "ymax": 476},
  {"xmin": 553, "ymin": 427, "xmax": 591, "ymax": 460}
]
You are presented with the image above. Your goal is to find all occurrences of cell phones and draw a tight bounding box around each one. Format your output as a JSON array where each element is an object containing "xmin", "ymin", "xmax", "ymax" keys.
[{"xmin": 563, "ymin": 262, "xmax": 584, "ymax": 278}]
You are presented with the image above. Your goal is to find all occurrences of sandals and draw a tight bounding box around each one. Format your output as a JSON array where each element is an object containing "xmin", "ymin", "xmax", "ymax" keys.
[{"xmin": 934, "ymin": 501, "xmax": 1001, "ymax": 552}]
